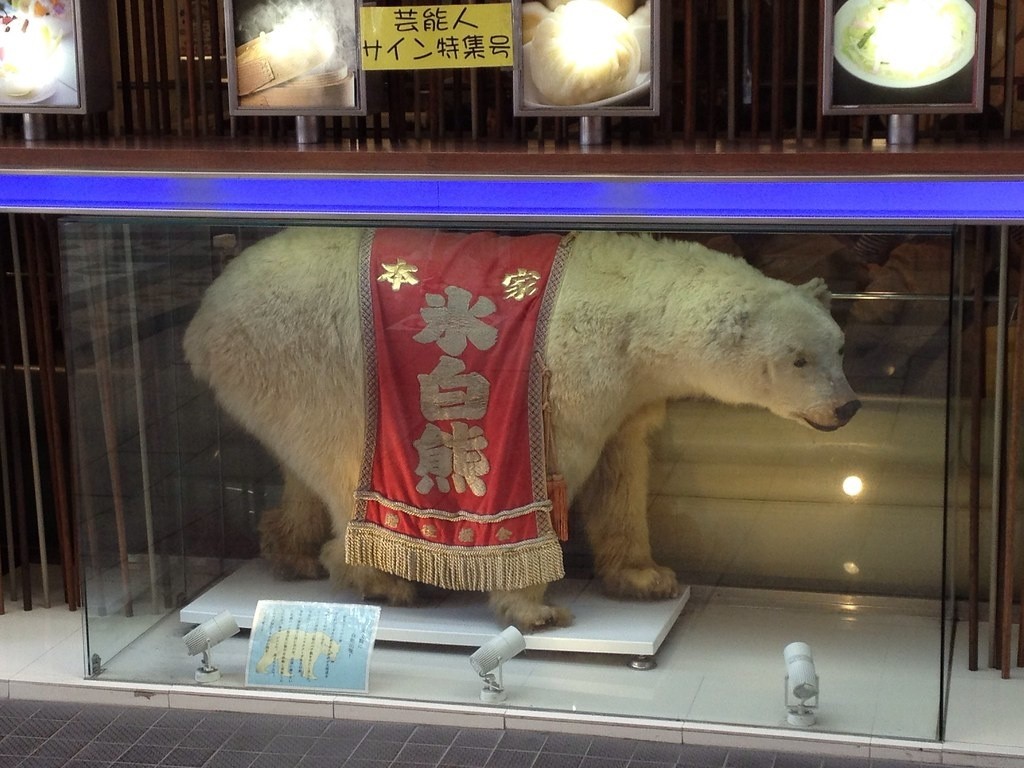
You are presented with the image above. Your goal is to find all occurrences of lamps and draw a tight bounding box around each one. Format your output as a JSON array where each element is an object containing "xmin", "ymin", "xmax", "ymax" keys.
[
  {"xmin": 181, "ymin": 610, "xmax": 241, "ymax": 685},
  {"xmin": 783, "ymin": 642, "xmax": 820, "ymax": 726},
  {"xmin": 469, "ymin": 626, "xmax": 526, "ymax": 702}
]
